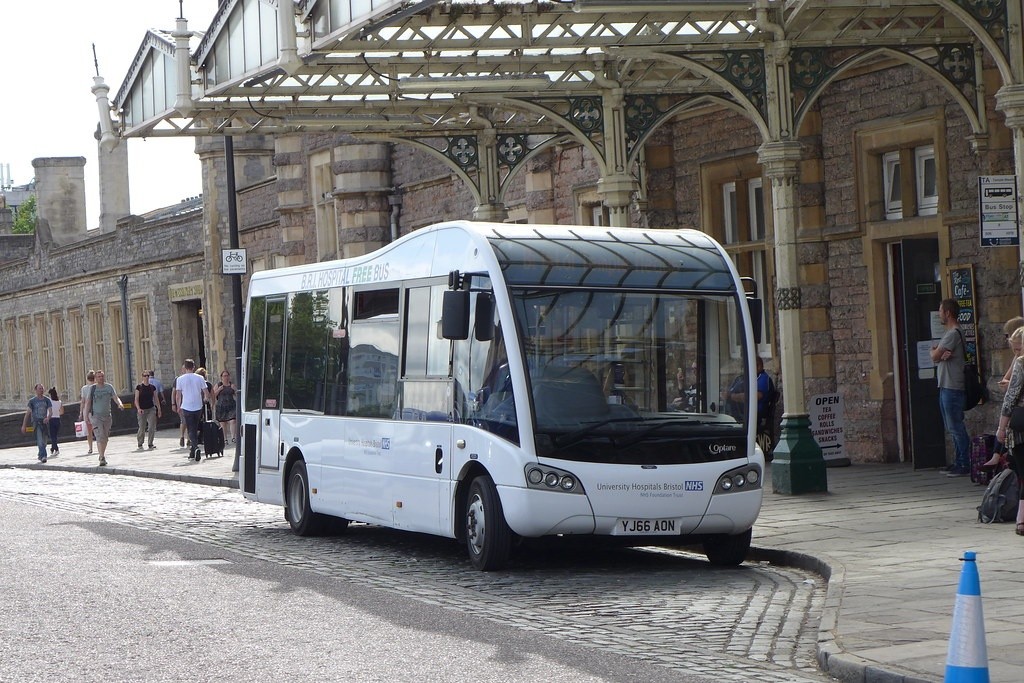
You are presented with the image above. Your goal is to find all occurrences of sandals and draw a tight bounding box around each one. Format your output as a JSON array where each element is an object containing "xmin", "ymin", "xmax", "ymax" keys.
[{"xmin": 1016, "ymin": 523, "xmax": 1024, "ymax": 535}]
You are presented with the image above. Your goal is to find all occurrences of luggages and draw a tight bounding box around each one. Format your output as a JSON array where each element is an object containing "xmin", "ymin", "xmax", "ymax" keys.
[
  {"xmin": 203, "ymin": 403, "xmax": 223, "ymax": 458},
  {"xmin": 971, "ymin": 435, "xmax": 995, "ymax": 482}
]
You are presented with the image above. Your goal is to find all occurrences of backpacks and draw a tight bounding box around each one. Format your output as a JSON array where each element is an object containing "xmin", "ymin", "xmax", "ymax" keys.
[{"xmin": 977, "ymin": 469, "xmax": 1020, "ymax": 524}]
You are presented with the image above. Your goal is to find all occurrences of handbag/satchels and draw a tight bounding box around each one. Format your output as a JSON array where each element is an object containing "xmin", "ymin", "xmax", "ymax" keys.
[
  {"xmin": 1009, "ymin": 407, "xmax": 1024, "ymax": 432},
  {"xmin": 75, "ymin": 421, "xmax": 88, "ymax": 437},
  {"xmin": 963, "ymin": 363, "xmax": 983, "ymax": 411}
]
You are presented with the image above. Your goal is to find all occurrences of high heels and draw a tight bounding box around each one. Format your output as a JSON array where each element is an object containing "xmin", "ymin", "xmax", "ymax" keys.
[{"xmin": 976, "ymin": 464, "xmax": 997, "ymax": 474}]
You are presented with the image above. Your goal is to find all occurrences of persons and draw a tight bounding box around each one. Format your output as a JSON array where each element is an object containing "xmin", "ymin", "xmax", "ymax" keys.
[
  {"xmin": 929, "ymin": 298, "xmax": 970, "ymax": 477},
  {"xmin": 976, "ymin": 316, "xmax": 1024, "ymax": 535},
  {"xmin": 674, "ymin": 356, "xmax": 775, "ymax": 462},
  {"xmin": 22, "ymin": 383, "xmax": 52, "ymax": 462},
  {"xmin": 195, "ymin": 367, "xmax": 215, "ymax": 445},
  {"xmin": 47, "ymin": 387, "xmax": 64, "ymax": 455},
  {"xmin": 147, "ymin": 370, "xmax": 166, "ymax": 431},
  {"xmin": 79, "ymin": 370, "xmax": 96, "ymax": 453},
  {"xmin": 214, "ymin": 370, "xmax": 236, "ymax": 444},
  {"xmin": 176, "ymin": 358, "xmax": 209, "ymax": 461},
  {"xmin": 85, "ymin": 370, "xmax": 124, "ymax": 466},
  {"xmin": 171, "ymin": 361, "xmax": 190, "ymax": 447},
  {"xmin": 135, "ymin": 370, "xmax": 161, "ymax": 449}
]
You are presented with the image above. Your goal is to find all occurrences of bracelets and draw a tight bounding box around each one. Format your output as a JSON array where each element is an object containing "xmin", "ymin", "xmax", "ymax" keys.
[{"xmin": 172, "ymin": 403, "xmax": 176, "ymax": 405}]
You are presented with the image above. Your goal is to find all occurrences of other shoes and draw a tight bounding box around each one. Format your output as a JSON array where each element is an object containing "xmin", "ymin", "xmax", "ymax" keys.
[
  {"xmin": 148, "ymin": 445, "xmax": 157, "ymax": 449},
  {"xmin": 224, "ymin": 440, "xmax": 229, "ymax": 444},
  {"xmin": 180, "ymin": 438, "xmax": 184, "ymax": 447},
  {"xmin": 190, "ymin": 449, "xmax": 195, "ymax": 458},
  {"xmin": 939, "ymin": 465, "xmax": 954, "ymax": 474},
  {"xmin": 945, "ymin": 469, "xmax": 971, "ymax": 476},
  {"xmin": 138, "ymin": 445, "xmax": 142, "ymax": 448},
  {"xmin": 38, "ymin": 456, "xmax": 47, "ymax": 463},
  {"xmin": 89, "ymin": 450, "xmax": 92, "ymax": 453},
  {"xmin": 232, "ymin": 439, "xmax": 236, "ymax": 443},
  {"xmin": 99, "ymin": 456, "xmax": 107, "ymax": 466},
  {"xmin": 195, "ymin": 449, "xmax": 201, "ymax": 461},
  {"xmin": 186, "ymin": 441, "xmax": 190, "ymax": 446}
]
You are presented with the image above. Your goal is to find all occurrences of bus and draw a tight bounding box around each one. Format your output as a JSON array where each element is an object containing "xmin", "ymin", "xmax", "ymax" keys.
[{"xmin": 238, "ymin": 217, "xmax": 767, "ymax": 570}]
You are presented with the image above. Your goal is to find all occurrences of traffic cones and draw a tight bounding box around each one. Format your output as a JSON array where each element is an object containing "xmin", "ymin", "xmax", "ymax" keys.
[{"xmin": 942, "ymin": 551, "xmax": 994, "ymax": 683}]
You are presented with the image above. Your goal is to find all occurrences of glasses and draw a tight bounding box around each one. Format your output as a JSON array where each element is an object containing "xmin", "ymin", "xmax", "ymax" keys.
[
  {"xmin": 1005, "ymin": 334, "xmax": 1010, "ymax": 338},
  {"xmin": 143, "ymin": 375, "xmax": 149, "ymax": 377}
]
